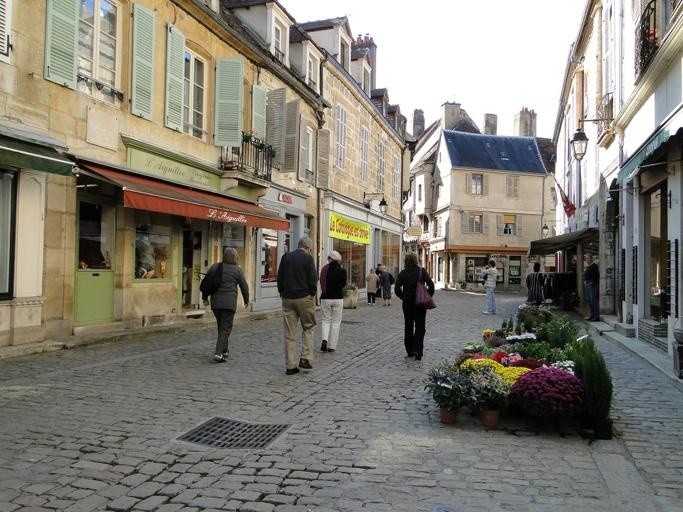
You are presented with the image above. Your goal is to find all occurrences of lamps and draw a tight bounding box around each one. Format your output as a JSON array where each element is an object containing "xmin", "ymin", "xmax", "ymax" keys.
[
  {"xmin": 570, "ymin": 117, "xmax": 614, "ymax": 161},
  {"xmin": 587, "ymin": 241, "xmax": 592, "ymax": 246},
  {"xmin": 541, "ymin": 223, "xmax": 548, "ymax": 238},
  {"xmin": 363, "ymin": 191, "xmax": 387, "ymax": 217}
]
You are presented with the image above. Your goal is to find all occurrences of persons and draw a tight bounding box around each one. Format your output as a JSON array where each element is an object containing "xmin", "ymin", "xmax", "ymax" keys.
[
  {"xmin": 394, "ymin": 251, "xmax": 435, "ymax": 361},
  {"xmin": 319, "ymin": 249, "xmax": 348, "ymax": 351},
  {"xmin": 483, "ymin": 260, "xmax": 498, "ymax": 315},
  {"xmin": 200, "ymin": 246, "xmax": 249, "ymax": 362},
  {"xmin": 278, "ymin": 236, "xmax": 318, "ymax": 375},
  {"xmin": 363, "ymin": 262, "xmax": 396, "ymax": 306},
  {"xmin": 525, "ymin": 263, "xmax": 546, "ymax": 307},
  {"xmin": 584, "ymin": 254, "xmax": 600, "ymax": 322}
]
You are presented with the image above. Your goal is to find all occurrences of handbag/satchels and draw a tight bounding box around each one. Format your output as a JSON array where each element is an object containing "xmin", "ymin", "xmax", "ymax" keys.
[{"xmin": 417, "ymin": 283, "xmax": 437, "ymax": 309}]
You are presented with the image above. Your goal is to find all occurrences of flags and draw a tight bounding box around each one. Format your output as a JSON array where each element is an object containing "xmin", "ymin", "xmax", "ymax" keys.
[{"xmin": 557, "ymin": 182, "xmax": 576, "ymax": 217}]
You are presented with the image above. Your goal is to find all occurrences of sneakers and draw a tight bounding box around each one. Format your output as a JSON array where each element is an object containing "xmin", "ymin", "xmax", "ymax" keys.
[
  {"xmin": 408, "ymin": 352, "xmax": 422, "ymax": 360},
  {"xmin": 383, "ymin": 303, "xmax": 390, "ymax": 306},
  {"xmin": 299, "ymin": 358, "xmax": 312, "ymax": 368},
  {"xmin": 321, "ymin": 341, "xmax": 334, "ymax": 352},
  {"xmin": 214, "ymin": 353, "xmax": 228, "ymax": 363},
  {"xmin": 287, "ymin": 367, "xmax": 299, "ymax": 375},
  {"xmin": 368, "ymin": 303, "xmax": 375, "ymax": 306}
]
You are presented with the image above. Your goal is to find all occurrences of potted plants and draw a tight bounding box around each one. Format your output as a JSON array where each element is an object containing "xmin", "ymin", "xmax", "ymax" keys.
[
  {"xmin": 465, "ymin": 364, "xmax": 509, "ymax": 430},
  {"xmin": 422, "ymin": 360, "xmax": 471, "ymax": 423},
  {"xmin": 511, "ymin": 367, "xmax": 584, "ymax": 433},
  {"xmin": 342, "ymin": 282, "xmax": 359, "ymax": 309}
]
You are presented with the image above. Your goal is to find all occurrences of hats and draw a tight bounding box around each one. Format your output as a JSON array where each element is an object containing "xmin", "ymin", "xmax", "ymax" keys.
[{"xmin": 329, "ymin": 251, "xmax": 341, "ymax": 261}]
